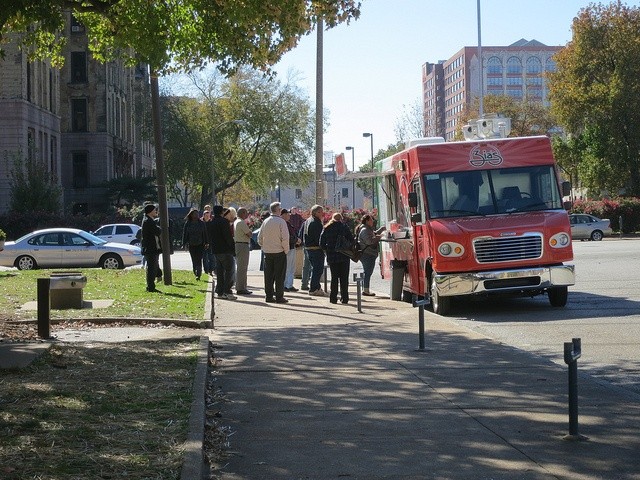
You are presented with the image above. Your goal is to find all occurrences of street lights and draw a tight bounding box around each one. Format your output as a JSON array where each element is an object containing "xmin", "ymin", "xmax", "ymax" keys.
[
  {"xmin": 345, "ymin": 146, "xmax": 355, "ymax": 211},
  {"xmin": 363, "ymin": 132, "xmax": 375, "ymax": 211},
  {"xmin": 328, "ymin": 164, "xmax": 336, "ymax": 209},
  {"xmin": 210, "ymin": 120, "xmax": 244, "ymax": 203}
]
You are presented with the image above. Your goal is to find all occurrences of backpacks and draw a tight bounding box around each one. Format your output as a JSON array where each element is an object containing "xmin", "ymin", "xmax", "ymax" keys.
[{"xmin": 350, "ymin": 226, "xmax": 373, "ymax": 262}]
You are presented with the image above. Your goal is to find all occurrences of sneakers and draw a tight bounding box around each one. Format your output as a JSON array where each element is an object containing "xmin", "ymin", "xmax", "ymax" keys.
[
  {"xmin": 155, "ymin": 276, "xmax": 162, "ymax": 283},
  {"xmin": 277, "ymin": 298, "xmax": 288, "ymax": 303},
  {"xmin": 309, "ymin": 288, "xmax": 329, "ymax": 297},
  {"xmin": 223, "ymin": 292, "xmax": 237, "ymax": 300},
  {"xmin": 301, "ymin": 286, "xmax": 309, "ymax": 290},
  {"xmin": 341, "ymin": 297, "xmax": 348, "ymax": 304},
  {"xmin": 196, "ymin": 275, "xmax": 200, "ymax": 280},
  {"xmin": 147, "ymin": 287, "xmax": 158, "ymax": 292},
  {"xmin": 217, "ymin": 293, "xmax": 223, "ymax": 299},
  {"xmin": 266, "ymin": 297, "xmax": 276, "ymax": 302}
]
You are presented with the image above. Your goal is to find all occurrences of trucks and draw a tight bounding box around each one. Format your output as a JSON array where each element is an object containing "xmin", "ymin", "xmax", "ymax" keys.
[{"xmin": 351, "ymin": 136, "xmax": 574, "ymax": 315}]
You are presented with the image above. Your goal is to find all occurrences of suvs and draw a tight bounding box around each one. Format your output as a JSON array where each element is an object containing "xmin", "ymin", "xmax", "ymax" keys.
[{"xmin": 92, "ymin": 224, "xmax": 142, "ymax": 246}]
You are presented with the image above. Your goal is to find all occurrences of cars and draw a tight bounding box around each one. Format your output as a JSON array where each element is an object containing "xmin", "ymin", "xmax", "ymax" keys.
[
  {"xmin": 569, "ymin": 213, "xmax": 612, "ymax": 241},
  {"xmin": 0, "ymin": 228, "xmax": 142, "ymax": 270}
]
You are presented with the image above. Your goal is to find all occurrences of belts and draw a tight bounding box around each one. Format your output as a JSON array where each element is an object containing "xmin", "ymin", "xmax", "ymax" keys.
[{"xmin": 236, "ymin": 241, "xmax": 249, "ymax": 244}]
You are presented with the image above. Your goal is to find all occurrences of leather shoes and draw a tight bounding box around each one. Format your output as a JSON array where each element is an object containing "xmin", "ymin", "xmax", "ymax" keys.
[
  {"xmin": 289, "ymin": 287, "xmax": 299, "ymax": 291},
  {"xmin": 362, "ymin": 292, "xmax": 376, "ymax": 296},
  {"xmin": 237, "ymin": 291, "xmax": 253, "ymax": 295},
  {"xmin": 284, "ymin": 287, "xmax": 289, "ymax": 292}
]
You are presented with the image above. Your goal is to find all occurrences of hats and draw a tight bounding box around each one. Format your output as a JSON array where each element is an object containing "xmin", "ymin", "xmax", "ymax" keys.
[
  {"xmin": 144, "ymin": 205, "xmax": 155, "ymax": 213},
  {"xmin": 281, "ymin": 208, "xmax": 291, "ymax": 215},
  {"xmin": 223, "ymin": 208, "xmax": 230, "ymax": 217}
]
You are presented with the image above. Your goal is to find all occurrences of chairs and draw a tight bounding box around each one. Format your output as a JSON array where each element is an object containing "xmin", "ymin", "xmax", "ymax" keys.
[{"xmin": 503, "ymin": 186, "xmax": 522, "ymax": 199}]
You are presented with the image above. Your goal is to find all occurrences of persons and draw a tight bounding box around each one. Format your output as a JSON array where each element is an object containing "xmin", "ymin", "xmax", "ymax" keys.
[
  {"xmin": 234, "ymin": 207, "xmax": 254, "ymax": 295},
  {"xmin": 140, "ymin": 205, "xmax": 162, "ymax": 292},
  {"xmin": 298, "ymin": 219, "xmax": 315, "ymax": 289},
  {"xmin": 303, "ymin": 204, "xmax": 324, "ymax": 296},
  {"xmin": 201, "ymin": 203, "xmax": 211, "ymax": 221},
  {"xmin": 318, "ymin": 213, "xmax": 353, "ymax": 304},
  {"xmin": 135, "ymin": 212, "xmax": 150, "ymax": 270},
  {"xmin": 212, "ymin": 205, "xmax": 237, "ymax": 300},
  {"xmin": 354, "ymin": 214, "xmax": 386, "ymax": 297},
  {"xmin": 280, "ymin": 209, "xmax": 299, "ymax": 292},
  {"xmin": 223, "ymin": 207, "xmax": 235, "ymax": 233},
  {"xmin": 257, "ymin": 202, "xmax": 289, "ymax": 303},
  {"xmin": 151, "ymin": 203, "xmax": 173, "ymax": 283},
  {"xmin": 181, "ymin": 207, "xmax": 209, "ymax": 281},
  {"xmin": 200, "ymin": 211, "xmax": 214, "ymax": 275},
  {"xmin": 290, "ymin": 206, "xmax": 302, "ymax": 230}
]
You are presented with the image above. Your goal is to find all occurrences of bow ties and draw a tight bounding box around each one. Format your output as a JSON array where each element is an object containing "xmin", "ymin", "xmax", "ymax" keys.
[{"xmin": 330, "ymin": 298, "xmax": 338, "ymax": 304}]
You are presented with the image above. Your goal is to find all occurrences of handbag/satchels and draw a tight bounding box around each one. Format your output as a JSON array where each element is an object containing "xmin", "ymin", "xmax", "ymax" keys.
[{"xmin": 336, "ymin": 224, "xmax": 354, "ymax": 258}]
